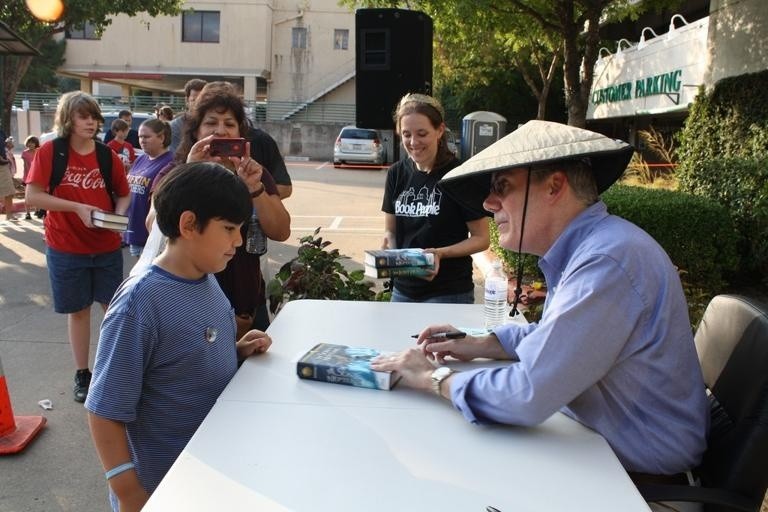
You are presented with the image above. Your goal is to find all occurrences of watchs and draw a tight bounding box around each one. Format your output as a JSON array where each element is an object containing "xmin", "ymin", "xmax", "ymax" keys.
[{"xmin": 429, "ymin": 366, "xmax": 460, "ymax": 398}]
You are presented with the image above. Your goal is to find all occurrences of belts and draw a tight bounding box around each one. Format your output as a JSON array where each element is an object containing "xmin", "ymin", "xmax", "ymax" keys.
[{"xmin": 654, "ymin": 469, "xmax": 699, "ymax": 487}]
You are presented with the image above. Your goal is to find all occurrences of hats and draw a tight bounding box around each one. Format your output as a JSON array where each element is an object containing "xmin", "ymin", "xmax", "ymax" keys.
[{"xmin": 438, "ymin": 119, "xmax": 636, "ymax": 216}]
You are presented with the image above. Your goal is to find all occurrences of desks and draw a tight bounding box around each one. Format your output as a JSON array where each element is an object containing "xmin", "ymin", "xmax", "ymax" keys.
[{"xmin": 140, "ymin": 298, "xmax": 654, "ymax": 512}]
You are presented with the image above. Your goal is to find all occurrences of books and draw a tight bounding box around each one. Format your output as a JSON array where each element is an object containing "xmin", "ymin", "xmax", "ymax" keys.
[
  {"xmin": 363, "ymin": 262, "xmax": 436, "ymax": 278},
  {"xmin": 91, "ymin": 209, "xmax": 130, "ymax": 231},
  {"xmin": 364, "ymin": 248, "xmax": 436, "ymax": 267},
  {"xmin": 297, "ymin": 343, "xmax": 403, "ymax": 391}
]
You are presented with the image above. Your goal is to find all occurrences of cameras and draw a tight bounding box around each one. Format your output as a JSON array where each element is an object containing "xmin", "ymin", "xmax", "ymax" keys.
[{"xmin": 209, "ymin": 138, "xmax": 245, "ymax": 158}]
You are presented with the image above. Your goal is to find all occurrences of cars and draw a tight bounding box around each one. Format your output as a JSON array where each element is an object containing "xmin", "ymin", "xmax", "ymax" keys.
[
  {"xmin": 332, "ymin": 125, "xmax": 387, "ymax": 166},
  {"xmin": 98, "ymin": 113, "xmax": 155, "ymax": 148}
]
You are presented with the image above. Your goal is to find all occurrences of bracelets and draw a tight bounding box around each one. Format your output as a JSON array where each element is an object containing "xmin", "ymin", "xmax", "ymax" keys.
[{"xmin": 105, "ymin": 458, "xmax": 137, "ymax": 481}]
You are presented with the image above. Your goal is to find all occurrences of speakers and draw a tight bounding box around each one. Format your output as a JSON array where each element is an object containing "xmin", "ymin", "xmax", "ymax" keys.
[{"xmin": 355, "ymin": 8, "xmax": 433, "ymax": 129}]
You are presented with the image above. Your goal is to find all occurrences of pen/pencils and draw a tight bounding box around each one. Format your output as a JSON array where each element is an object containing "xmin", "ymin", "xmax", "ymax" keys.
[{"xmin": 411, "ymin": 332, "xmax": 467, "ymax": 340}]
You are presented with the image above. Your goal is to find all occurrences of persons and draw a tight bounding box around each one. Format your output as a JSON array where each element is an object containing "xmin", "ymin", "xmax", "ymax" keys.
[
  {"xmin": 82, "ymin": 162, "xmax": 273, "ymax": 512},
  {"xmin": 169, "ymin": 80, "xmax": 292, "ymax": 332},
  {"xmin": 3, "ymin": 130, "xmax": 46, "ymax": 222},
  {"xmin": 380, "ymin": 93, "xmax": 492, "ymax": 304},
  {"xmin": 104, "ymin": 78, "xmax": 205, "ymax": 260},
  {"xmin": 25, "ymin": 90, "xmax": 131, "ymax": 403},
  {"xmin": 368, "ymin": 120, "xmax": 710, "ymax": 512}
]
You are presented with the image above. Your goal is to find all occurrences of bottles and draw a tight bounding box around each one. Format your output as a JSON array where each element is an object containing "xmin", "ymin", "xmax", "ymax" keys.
[{"xmin": 483, "ymin": 261, "xmax": 509, "ymax": 333}]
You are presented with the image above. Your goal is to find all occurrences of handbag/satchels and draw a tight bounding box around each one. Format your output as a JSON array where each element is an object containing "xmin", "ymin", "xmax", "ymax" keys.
[
  {"xmin": 235, "ymin": 317, "xmax": 254, "ymax": 343},
  {"xmin": 245, "ymin": 215, "xmax": 268, "ymax": 257}
]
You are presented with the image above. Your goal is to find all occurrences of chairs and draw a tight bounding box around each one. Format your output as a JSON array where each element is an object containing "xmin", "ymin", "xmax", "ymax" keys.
[{"xmin": 630, "ymin": 293, "xmax": 768, "ymax": 512}]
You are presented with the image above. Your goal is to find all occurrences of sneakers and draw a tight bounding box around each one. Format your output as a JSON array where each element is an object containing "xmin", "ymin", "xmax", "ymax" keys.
[{"xmin": 73, "ymin": 370, "xmax": 92, "ymax": 402}]
